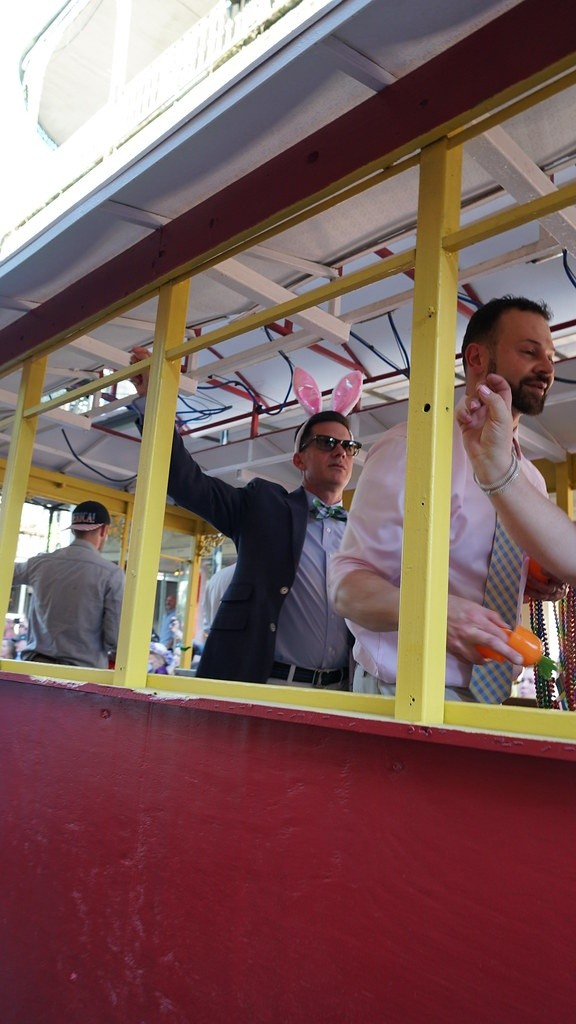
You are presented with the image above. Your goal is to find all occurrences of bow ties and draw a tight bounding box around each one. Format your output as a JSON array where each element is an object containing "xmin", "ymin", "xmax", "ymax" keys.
[{"xmin": 309, "ymin": 498, "xmax": 347, "ymax": 522}]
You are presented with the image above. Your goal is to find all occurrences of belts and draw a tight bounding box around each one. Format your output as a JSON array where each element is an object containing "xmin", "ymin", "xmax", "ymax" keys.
[
  {"xmin": 32, "ymin": 654, "xmax": 53, "ymax": 663},
  {"xmin": 269, "ymin": 661, "xmax": 349, "ymax": 688}
]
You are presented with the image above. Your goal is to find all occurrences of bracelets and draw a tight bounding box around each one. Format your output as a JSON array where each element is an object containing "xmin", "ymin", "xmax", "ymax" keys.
[{"xmin": 470, "ymin": 453, "xmax": 520, "ymax": 497}]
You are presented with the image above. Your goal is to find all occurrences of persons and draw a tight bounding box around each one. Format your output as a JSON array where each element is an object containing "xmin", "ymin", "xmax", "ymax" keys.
[
  {"xmin": 126, "ymin": 345, "xmax": 359, "ymax": 693},
  {"xmin": 1, "ymin": 620, "xmax": 29, "ymax": 662},
  {"xmin": 11, "ymin": 497, "xmax": 122, "ymax": 670},
  {"xmin": 330, "ymin": 292, "xmax": 557, "ymax": 705},
  {"xmin": 148, "ymin": 594, "xmax": 183, "ymax": 674},
  {"xmin": 456, "ymin": 371, "xmax": 576, "ymax": 584}
]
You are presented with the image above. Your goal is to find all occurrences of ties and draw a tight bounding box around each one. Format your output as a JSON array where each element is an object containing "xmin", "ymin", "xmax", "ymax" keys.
[{"xmin": 468, "ymin": 442, "xmax": 524, "ymax": 704}]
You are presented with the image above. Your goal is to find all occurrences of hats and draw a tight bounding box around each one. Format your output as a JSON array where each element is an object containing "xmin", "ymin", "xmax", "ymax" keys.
[{"xmin": 66, "ymin": 501, "xmax": 110, "ymax": 531}]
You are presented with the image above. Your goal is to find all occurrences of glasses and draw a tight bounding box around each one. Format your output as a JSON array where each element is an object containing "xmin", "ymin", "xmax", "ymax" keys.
[{"xmin": 300, "ymin": 434, "xmax": 362, "ymax": 456}]
